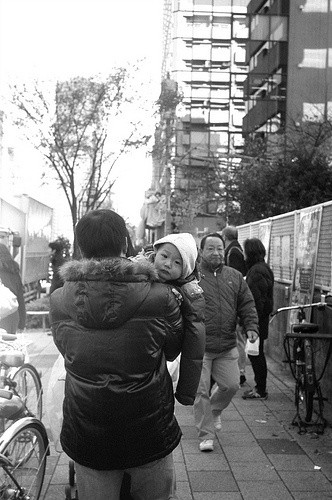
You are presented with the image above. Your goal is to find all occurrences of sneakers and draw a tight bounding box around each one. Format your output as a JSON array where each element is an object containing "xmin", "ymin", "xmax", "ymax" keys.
[
  {"xmin": 198, "ymin": 439, "xmax": 214, "ymax": 452},
  {"xmin": 214, "ymin": 414, "xmax": 222, "ymax": 431},
  {"xmin": 242, "ymin": 387, "xmax": 269, "ymax": 400}
]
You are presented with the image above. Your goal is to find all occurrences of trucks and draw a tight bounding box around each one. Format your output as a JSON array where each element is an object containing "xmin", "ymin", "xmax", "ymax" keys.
[{"xmin": 0, "ymin": 192, "xmax": 55, "ymax": 303}]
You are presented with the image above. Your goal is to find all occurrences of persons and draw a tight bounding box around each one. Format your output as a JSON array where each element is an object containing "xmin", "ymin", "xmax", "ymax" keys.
[
  {"xmin": 242, "ymin": 238, "xmax": 279, "ymax": 399},
  {"xmin": 0, "ymin": 243, "xmax": 27, "ymax": 336},
  {"xmin": 48, "ymin": 243, "xmax": 64, "ymax": 294},
  {"xmin": 193, "ymin": 234, "xmax": 260, "ymax": 452},
  {"xmin": 50, "ymin": 209, "xmax": 184, "ymax": 500},
  {"xmin": 221, "ymin": 226, "xmax": 248, "ymax": 386},
  {"xmin": 129, "ymin": 231, "xmax": 206, "ymax": 406}
]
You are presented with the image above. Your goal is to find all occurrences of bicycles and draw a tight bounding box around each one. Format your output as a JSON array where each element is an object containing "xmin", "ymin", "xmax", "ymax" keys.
[
  {"xmin": 270, "ymin": 301, "xmax": 332, "ymax": 434},
  {"xmin": 0, "ymin": 386, "xmax": 54, "ymax": 500},
  {"xmin": 0, "ymin": 327, "xmax": 21, "ymax": 354},
  {"xmin": 0, "ymin": 352, "xmax": 44, "ymax": 420}
]
